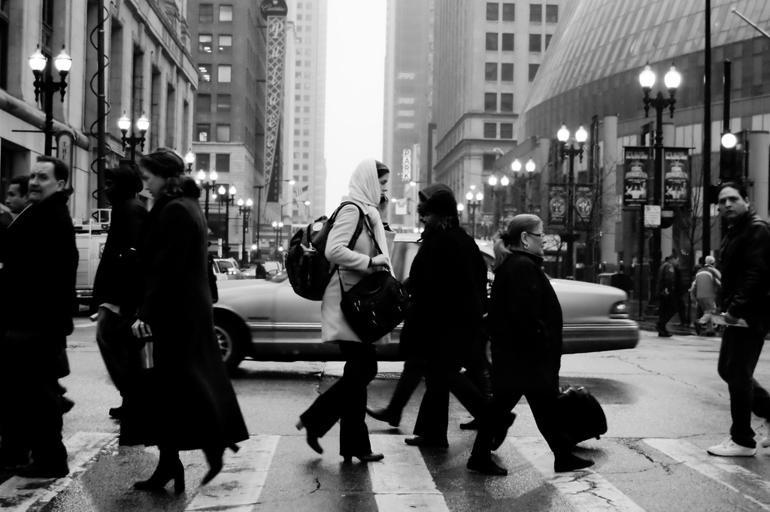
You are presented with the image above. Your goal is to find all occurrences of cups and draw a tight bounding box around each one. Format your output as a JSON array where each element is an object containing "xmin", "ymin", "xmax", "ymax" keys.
[{"xmin": 134, "ymin": 332, "xmax": 157, "ymax": 371}]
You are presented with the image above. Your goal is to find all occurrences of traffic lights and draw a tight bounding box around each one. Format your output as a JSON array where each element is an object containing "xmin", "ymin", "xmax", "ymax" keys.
[{"xmin": 721, "ymin": 132, "xmax": 739, "ymax": 181}]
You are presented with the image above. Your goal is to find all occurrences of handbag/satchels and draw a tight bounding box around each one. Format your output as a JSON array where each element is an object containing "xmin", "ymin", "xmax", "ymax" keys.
[{"xmin": 340, "ymin": 270, "xmax": 414, "ymax": 343}]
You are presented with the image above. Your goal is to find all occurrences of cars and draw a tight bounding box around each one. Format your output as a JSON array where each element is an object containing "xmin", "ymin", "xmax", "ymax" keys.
[
  {"xmin": 207, "ymin": 253, "xmax": 283, "ymax": 283},
  {"xmin": 213, "ymin": 233, "xmax": 640, "ymax": 387}
]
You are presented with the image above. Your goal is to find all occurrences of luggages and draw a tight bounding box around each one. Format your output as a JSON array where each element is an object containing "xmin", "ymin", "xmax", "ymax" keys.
[{"xmin": 557, "ymin": 384, "xmax": 607, "ymax": 443}]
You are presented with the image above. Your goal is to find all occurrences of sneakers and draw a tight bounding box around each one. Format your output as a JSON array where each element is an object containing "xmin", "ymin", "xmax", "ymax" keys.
[
  {"xmin": 707, "ymin": 437, "xmax": 757, "ymax": 456},
  {"xmin": 15, "ymin": 463, "xmax": 68, "ymax": 478}
]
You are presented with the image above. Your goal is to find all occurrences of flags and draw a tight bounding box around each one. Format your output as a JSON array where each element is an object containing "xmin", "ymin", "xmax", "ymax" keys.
[
  {"xmin": 661, "ymin": 146, "xmax": 695, "ymax": 211},
  {"xmin": 573, "ymin": 183, "xmax": 598, "ymax": 232},
  {"xmin": 546, "ymin": 182, "xmax": 570, "ymax": 232},
  {"xmin": 623, "ymin": 146, "xmax": 650, "ymax": 209}
]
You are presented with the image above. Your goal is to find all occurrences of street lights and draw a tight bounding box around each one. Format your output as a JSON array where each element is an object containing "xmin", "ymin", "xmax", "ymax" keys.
[
  {"xmin": 28, "ymin": 43, "xmax": 73, "ymax": 158},
  {"xmin": 639, "ymin": 57, "xmax": 680, "ymax": 315},
  {"xmin": 198, "ymin": 167, "xmax": 218, "ymax": 224},
  {"xmin": 168, "ymin": 145, "xmax": 195, "ymax": 179},
  {"xmin": 237, "ymin": 197, "xmax": 254, "ymax": 263},
  {"xmin": 214, "ymin": 185, "xmax": 236, "ymax": 252},
  {"xmin": 116, "ymin": 108, "xmax": 153, "ymax": 161},
  {"xmin": 510, "ymin": 153, "xmax": 535, "ymax": 220},
  {"xmin": 250, "ymin": 178, "xmax": 312, "ymax": 263},
  {"xmin": 389, "ymin": 172, "xmax": 509, "ymax": 243},
  {"xmin": 556, "ymin": 123, "xmax": 590, "ymax": 278}
]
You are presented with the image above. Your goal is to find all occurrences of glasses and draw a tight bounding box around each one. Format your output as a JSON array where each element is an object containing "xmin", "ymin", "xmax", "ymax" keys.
[{"xmin": 528, "ymin": 231, "xmax": 543, "ymax": 237}]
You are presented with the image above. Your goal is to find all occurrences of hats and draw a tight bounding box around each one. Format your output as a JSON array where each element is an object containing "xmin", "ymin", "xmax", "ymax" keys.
[{"xmin": 142, "ymin": 151, "xmax": 184, "ymax": 177}]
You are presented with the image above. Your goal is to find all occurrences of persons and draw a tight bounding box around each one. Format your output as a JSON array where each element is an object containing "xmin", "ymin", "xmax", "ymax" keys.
[
  {"xmin": 403, "ymin": 183, "xmax": 516, "ymax": 453},
  {"xmin": 1, "ymin": 153, "xmax": 80, "ymax": 480},
  {"xmin": 365, "ymin": 361, "xmax": 512, "ymax": 432},
  {"xmin": 89, "ymin": 159, "xmax": 150, "ymax": 421},
  {"xmin": 464, "ymin": 212, "xmax": 596, "ymax": 477},
  {"xmin": 296, "ymin": 158, "xmax": 396, "ymax": 470},
  {"xmin": 652, "ymin": 253, "xmax": 722, "ymax": 339},
  {"xmin": 127, "ymin": 142, "xmax": 250, "ymax": 497},
  {"xmin": 705, "ymin": 178, "xmax": 770, "ymax": 459}
]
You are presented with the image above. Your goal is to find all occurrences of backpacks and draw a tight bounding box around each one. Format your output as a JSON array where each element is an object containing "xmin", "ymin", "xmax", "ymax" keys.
[{"xmin": 285, "ymin": 201, "xmax": 365, "ymax": 300}]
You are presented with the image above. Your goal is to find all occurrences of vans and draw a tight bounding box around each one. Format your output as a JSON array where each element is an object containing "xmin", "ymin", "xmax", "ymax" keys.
[{"xmin": 72, "ymin": 208, "xmax": 111, "ymax": 318}]
